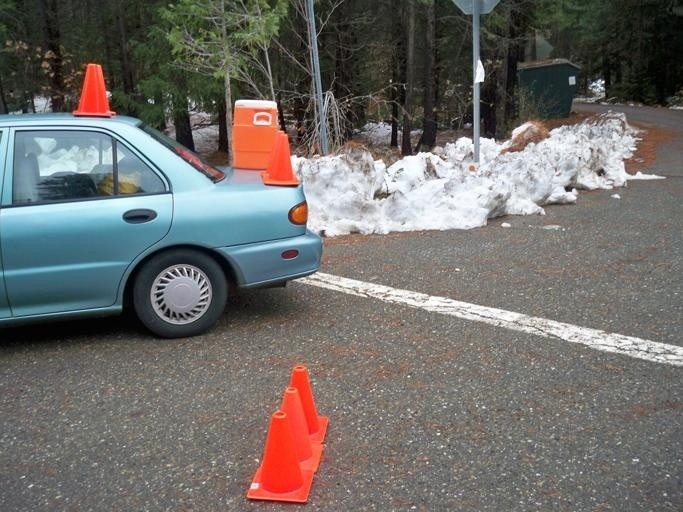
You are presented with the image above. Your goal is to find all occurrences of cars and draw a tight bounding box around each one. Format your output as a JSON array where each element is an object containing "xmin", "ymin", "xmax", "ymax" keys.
[{"xmin": 0, "ymin": 108, "xmax": 322, "ymax": 353}]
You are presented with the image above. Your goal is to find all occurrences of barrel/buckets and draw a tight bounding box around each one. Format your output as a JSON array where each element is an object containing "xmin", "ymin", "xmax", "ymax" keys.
[{"xmin": 231, "ymin": 100, "xmax": 278, "ymax": 170}]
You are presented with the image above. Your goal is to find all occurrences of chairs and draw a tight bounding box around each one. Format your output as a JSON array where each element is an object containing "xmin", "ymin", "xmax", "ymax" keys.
[{"xmin": 14, "ymin": 153, "xmax": 40, "ymax": 203}]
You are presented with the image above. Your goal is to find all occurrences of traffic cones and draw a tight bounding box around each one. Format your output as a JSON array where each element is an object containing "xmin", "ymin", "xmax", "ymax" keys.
[
  {"xmin": 270, "ymin": 383, "xmax": 319, "ymax": 464},
  {"xmin": 261, "ymin": 126, "xmax": 300, "ymax": 188},
  {"xmin": 246, "ymin": 406, "xmax": 315, "ymax": 505},
  {"xmin": 282, "ymin": 363, "xmax": 329, "ymax": 435},
  {"xmin": 72, "ymin": 61, "xmax": 117, "ymax": 120}
]
